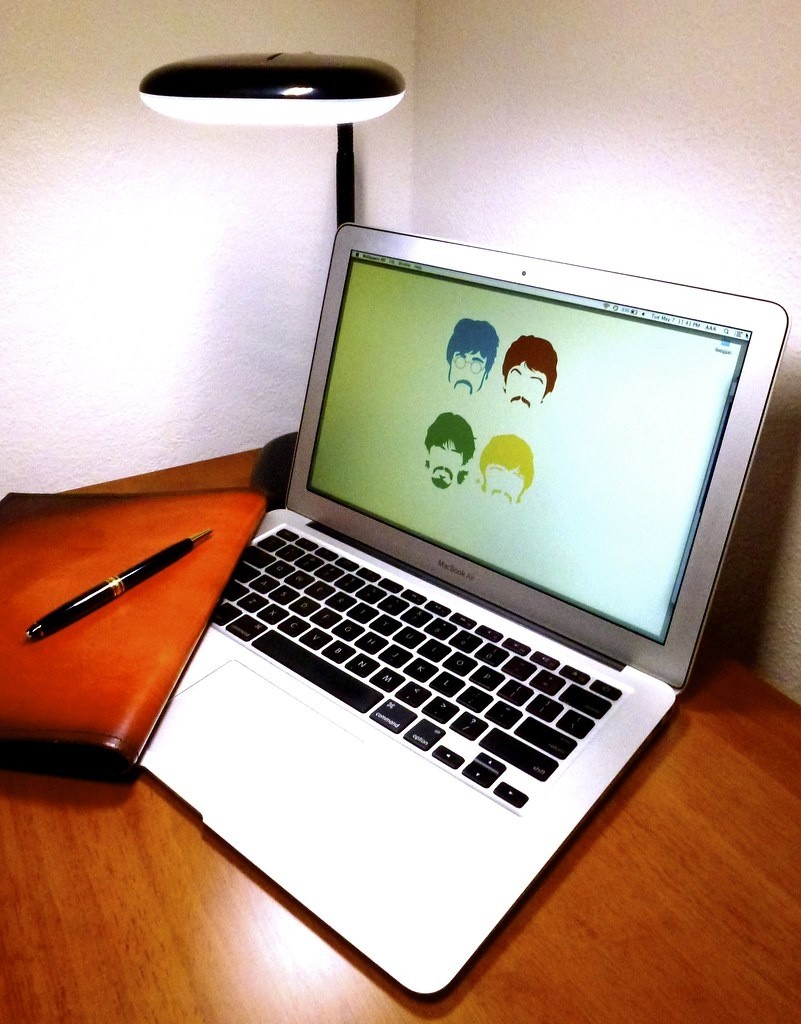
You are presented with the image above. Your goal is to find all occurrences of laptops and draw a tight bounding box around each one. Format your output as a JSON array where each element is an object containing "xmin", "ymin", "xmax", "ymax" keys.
[{"xmin": 137, "ymin": 222, "xmax": 792, "ymax": 997}]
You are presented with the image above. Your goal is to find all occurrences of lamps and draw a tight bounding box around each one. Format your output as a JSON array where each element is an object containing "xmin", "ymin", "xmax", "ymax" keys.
[{"xmin": 138, "ymin": 52, "xmax": 405, "ymax": 509}]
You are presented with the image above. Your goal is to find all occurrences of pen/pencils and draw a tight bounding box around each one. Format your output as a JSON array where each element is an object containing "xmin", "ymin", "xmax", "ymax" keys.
[{"xmin": 25, "ymin": 528, "xmax": 216, "ymax": 656}]
